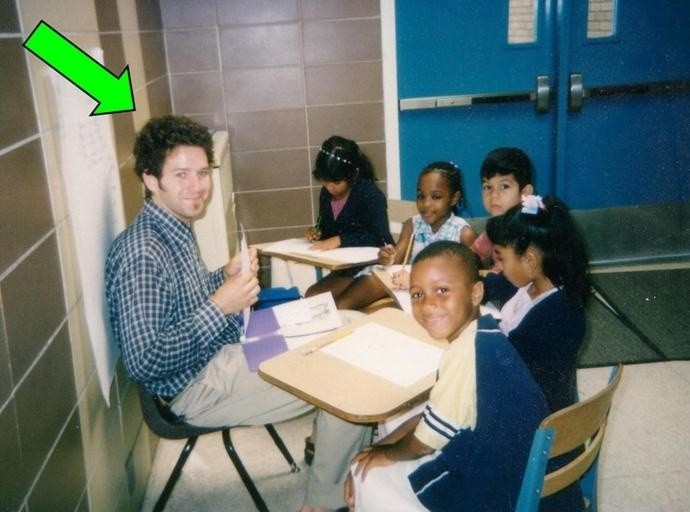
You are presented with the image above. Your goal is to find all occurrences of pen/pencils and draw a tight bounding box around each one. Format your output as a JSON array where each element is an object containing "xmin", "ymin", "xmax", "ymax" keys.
[
  {"xmin": 380, "ymin": 234, "xmax": 396, "ymax": 259},
  {"xmin": 311, "ymin": 214, "xmax": 325, "ymax": 242}
]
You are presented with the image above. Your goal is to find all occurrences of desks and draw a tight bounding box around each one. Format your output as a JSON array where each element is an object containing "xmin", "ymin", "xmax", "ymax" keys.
[
  {"xmin": 249, "ymin": 235, "xmax": 387, "ymax": 289},
  {"xmin": 371, "ymin": 264, "xmax": 502, "ymax": 321},
  {"xmin": 257, "ymin": 306, "xmax": 450, "ymax": 427}
]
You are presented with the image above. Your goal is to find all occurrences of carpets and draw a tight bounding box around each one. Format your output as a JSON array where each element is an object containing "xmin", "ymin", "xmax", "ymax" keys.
[{"xmin": 569, "ymin": 201, "xmax": 689, "ymax": 369}]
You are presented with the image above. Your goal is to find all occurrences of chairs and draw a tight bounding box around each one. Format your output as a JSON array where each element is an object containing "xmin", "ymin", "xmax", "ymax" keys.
[
  {"xmin": 358, "ymin": 198, "xmax": 425, "ymax": 315},
  {"xmin": 512, "ymin": 361, "xmax": 625, "ymax": 512},
  {"xmin": 137, "ymin": 384, "xmax": 301, "ymax": 511}
]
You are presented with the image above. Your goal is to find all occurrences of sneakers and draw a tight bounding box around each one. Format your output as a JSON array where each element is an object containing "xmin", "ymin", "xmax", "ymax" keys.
[{"xmin": 304, "ymin": 436, "xmax": 315, "ymax": 466}]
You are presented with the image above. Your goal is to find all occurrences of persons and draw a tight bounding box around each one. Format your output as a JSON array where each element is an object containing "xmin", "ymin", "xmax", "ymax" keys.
[
  {"xmin": 362, "ymin": 159, "xmax": 477, "ymax": 318},
  {"xmin": 480, "ymin": 189, "xmax": 594, "ymax": 479},
  {"xmin": 101, "ymin": 116, "xmax": 376, "ymax": 512},
  {"xmin": 391, "ymin": 144, "xmax": 538, "ymax": 293},
  {"xmin": 342, "ymin": 238, "xmax": 588, "ymax": 512},
  {"xmin": 304, "ymin": 135, "xmax": 397, "ymax": 315}
]
